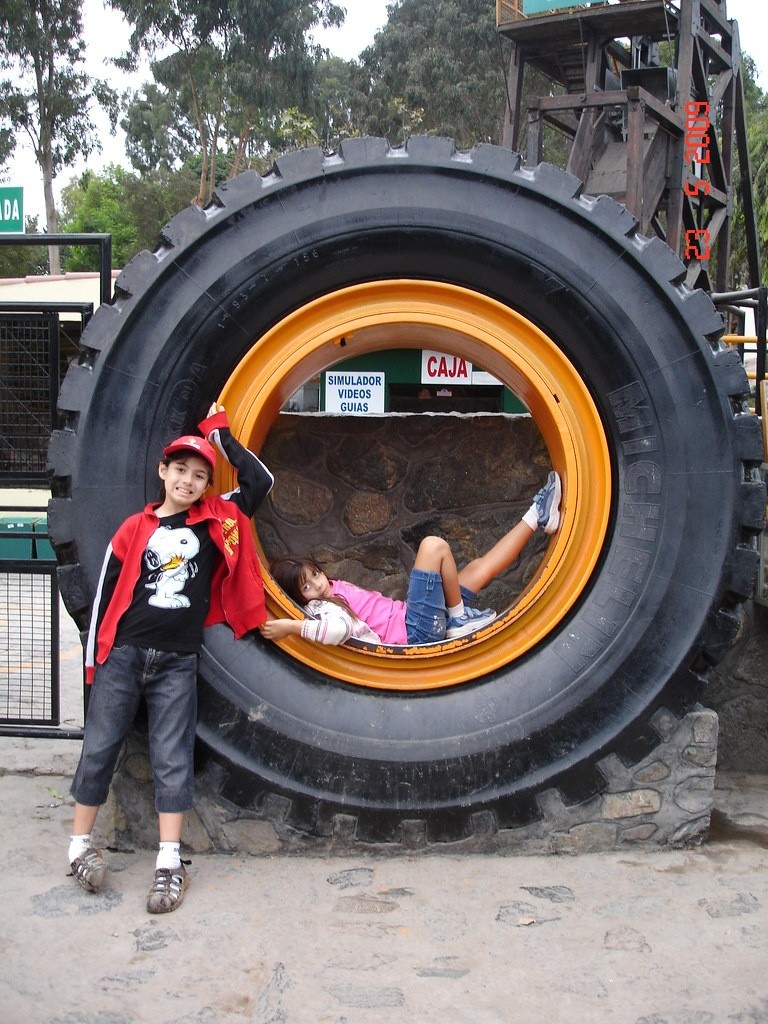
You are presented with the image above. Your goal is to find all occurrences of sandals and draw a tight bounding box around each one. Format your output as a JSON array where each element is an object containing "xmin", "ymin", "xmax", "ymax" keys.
[
  {"xmin": 66, "ymin": 846, "xmax": 107, "ymax": 893},
  {"xmin": 147, "ymin": 858, "xmax": 192, "ymax": 914}
]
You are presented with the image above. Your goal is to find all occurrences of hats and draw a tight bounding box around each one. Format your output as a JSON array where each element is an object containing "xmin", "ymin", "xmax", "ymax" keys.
[{"xmin": 162, "ymin": 436, "xmax": 216, "ymax": 472}]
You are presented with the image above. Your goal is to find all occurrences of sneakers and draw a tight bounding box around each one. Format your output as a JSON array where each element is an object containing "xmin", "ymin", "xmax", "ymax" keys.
[
  {"xmin": 532, "ymin": 471, "xmax": 561, "ymax": 535},
  {"xmin": 445, "ymin": 607, "xmax": 496, "ymax": 639}
]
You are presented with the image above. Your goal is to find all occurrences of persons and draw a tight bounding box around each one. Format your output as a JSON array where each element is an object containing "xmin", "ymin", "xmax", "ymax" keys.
[
  {"xmin": 260, "ymin": 471, "xmax": 564, "ymax": 647},
  {"xmin": 68, "ymin": 402, "xmax": 274, "ymax": 915}
]
